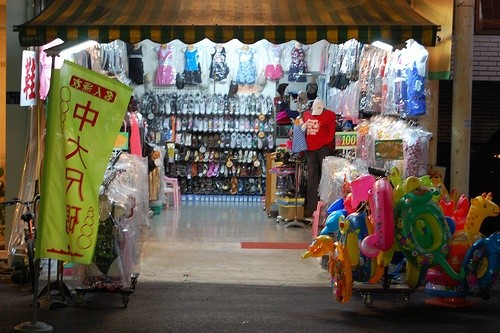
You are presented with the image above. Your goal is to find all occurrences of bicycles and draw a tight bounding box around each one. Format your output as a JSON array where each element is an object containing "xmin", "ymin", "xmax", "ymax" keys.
[{"xmin": 0, "ymin": 194, "xmax": 41, "ymax": 292}]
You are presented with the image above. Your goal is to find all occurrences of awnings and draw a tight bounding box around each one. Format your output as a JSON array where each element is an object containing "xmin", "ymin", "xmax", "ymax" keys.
[{"xmin": 13, "ymin": 0, "xmax": 447, "ymax": 56}]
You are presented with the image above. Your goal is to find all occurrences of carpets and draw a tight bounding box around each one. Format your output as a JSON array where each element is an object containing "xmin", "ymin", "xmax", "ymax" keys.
[{"xmin": 241, "ymin": 241, "xmax": 311, "ymax": 249}]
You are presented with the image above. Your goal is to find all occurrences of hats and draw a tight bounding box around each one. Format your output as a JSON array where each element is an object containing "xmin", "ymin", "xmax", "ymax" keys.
[
  {"xmin": 255, "ymin": 74, "xmax": 267, "ymax": 91},
  {"xmin": 176, "ymin": 73, "xmax": 185, "ymax": 89},
  {"xmin": 311, "ymin": 99, "xmax": 325, "ymax": 115},
  {"xmin": 297, "ymin": 90, "xmax": 308, "ymax": 104},
  {"xmin": 226, "ymin": 80, "xmax": 238, "ymax": 98},
  {"xmin": 277, "ymin": 83, "xmax": 290, "ymax": 99},
  {"xmin": 306, "ymin": 83, "xmax": 318, "ymax": 99}
]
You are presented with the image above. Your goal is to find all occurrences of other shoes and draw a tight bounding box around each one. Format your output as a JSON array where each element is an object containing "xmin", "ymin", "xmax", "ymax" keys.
[{"xmin": 140, "ymin": 90, "xmax": 276, "ymax": 194}]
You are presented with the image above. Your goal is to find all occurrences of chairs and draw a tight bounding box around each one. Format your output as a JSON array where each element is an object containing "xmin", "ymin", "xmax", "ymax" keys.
[{"xmin": 164, "ymin": 178, "xmax": 182, "ymax": 207}]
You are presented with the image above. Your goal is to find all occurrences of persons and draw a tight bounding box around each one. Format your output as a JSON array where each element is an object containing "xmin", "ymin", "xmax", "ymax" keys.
[
  {"xmin": 303, "ymin": 103, "xmax": 335, "ymax": 216},
  {"xmin": 124, "ymin": 39, "xmax": 432, "ymax": 114},
  {"xmin": 357, "ymin": 117, "xmax": 436, "ymax": 180},
  {"xmin": 293, "ymin": 116, "xmax": 307, "ymax": 151},
  {"xmin": 84, "ymin": 180, "xmax": 141, "ymax": 280}
]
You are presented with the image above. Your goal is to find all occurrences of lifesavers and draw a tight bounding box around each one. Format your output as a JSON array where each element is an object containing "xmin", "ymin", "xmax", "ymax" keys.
[{"xmin": 301, "ymin": 165, "xmax": 499, "ymax": 306}]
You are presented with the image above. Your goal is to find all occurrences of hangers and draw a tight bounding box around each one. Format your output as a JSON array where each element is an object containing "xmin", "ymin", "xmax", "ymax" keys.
[{"xmin": 133, "ymin": 42, "xmax": 304, "ymax": 51}]
